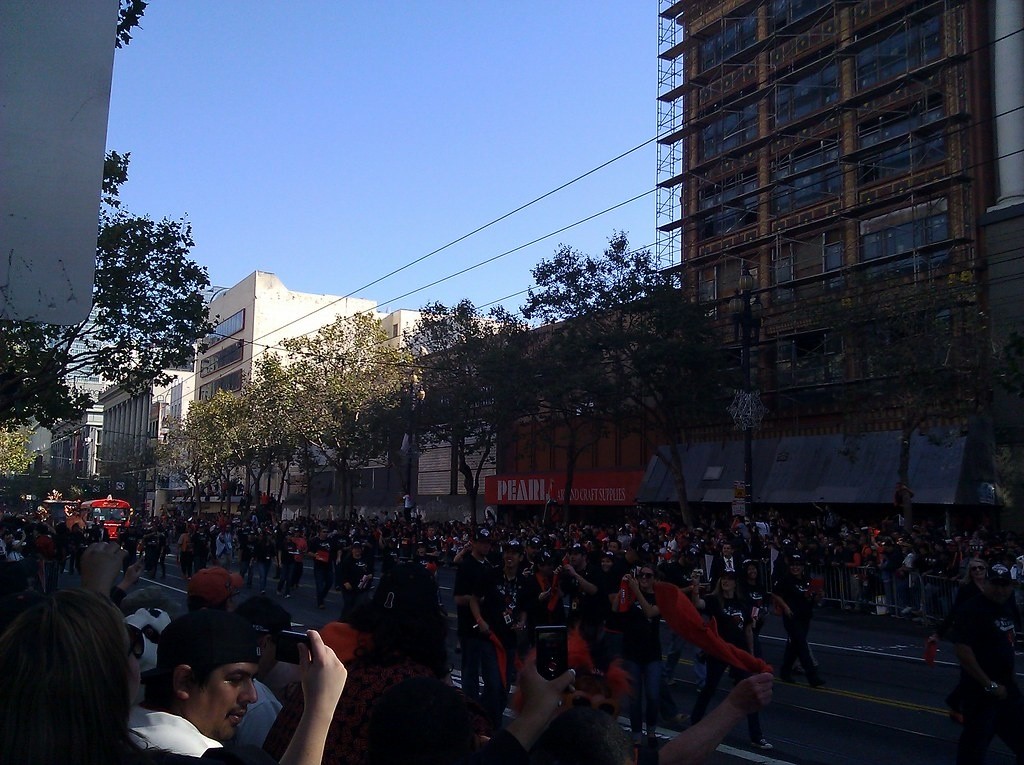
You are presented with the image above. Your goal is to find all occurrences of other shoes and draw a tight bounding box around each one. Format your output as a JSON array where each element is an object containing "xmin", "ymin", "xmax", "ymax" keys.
[
  {"xmin": 950, "ymin": 710, "xmax": 964, "ymax": 724},
  {"xmin": 815, "ymin": 681, "xmax": 836, "ymax": 689},
  {"xmin": 662, "ymin": 713, "xmax": 690, "ymax": 723},
  {"xmin": 319, "ymin": 604, "xmax": 325, "ymax": 609},
  {"xmin": 844, "ymin": 603, "xmax": 930, "ymax": 625},
  {"xmin": 647, "ymin": 734, "xmax": 658, "ymax": 749}
]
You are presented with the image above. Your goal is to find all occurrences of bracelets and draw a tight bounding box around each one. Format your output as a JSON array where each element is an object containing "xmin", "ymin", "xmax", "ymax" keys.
[
  {"xmin": 692, "ymin": 592, "xmax": 699, "ymax": 595},
  {"xmin": 476, "ymin": 616, "xmax": 483, "ymax": 626}
]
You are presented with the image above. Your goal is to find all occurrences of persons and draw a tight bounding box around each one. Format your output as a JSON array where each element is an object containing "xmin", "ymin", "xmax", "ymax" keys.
[
  {"xmin": 0, "ymin": 587, "xmax": 348, "ymax": 765},
  {"xmin": 0, "ymin": 489, "xmax": 1024, "ymax": 765},
  {"xmin": 199, "ymin": 479, "xmax": 221, "ymax": 502},
  {"xmin": 237, "ymin": 482, "xmax": 244, "ymax": 495},
  {"xmin": 161, "ymin": 476, "xmax": 173, "ymax": 488},
  {"xmin": 183, "ymin": 487, "xmax": 193, "ymax": 502},
  {"xmin": 217, "ymin": 482, "xmax": 228, "ymax": 501}
]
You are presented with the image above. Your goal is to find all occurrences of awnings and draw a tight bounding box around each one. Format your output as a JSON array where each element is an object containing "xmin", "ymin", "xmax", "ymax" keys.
[{"xmin": 634, "ymin": 425, "xmax": 996, "ymax": 506}]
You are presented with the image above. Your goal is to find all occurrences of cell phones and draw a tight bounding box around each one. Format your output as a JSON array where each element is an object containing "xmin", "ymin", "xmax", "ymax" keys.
[{"xmin": 631, "ymin": 569, "xmax": 637, "ymax": 579}]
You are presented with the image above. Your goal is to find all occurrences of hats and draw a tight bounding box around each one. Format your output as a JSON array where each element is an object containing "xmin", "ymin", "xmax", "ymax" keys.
[
  {"xmin": 345, "ymin": 524, "xmax": 805, "ymax": 576},
  {"xmin": 156, "ymin": 609, "xmax": 261, "ymax": 679},
  {"xmin": 189, "ymin": 568, "xmax": 242, "ymax": 601},
  {"xmin": 233, "ymin": 591, "xmax": 305, "ymax": 632}
]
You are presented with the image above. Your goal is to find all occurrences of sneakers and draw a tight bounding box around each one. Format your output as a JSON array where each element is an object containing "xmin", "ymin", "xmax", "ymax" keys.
[{"xmin": 749, "ymin": 737, "xmax": 772, "ymax": 750}]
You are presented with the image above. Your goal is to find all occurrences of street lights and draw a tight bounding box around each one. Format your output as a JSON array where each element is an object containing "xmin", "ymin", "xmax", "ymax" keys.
[
  {"xmin": 726, "ymin": 263, "xmax": 764, "ymax": 526},
  {"xmin": 408, "ymin": 372, "xmax": 425, "ymax": 493}
]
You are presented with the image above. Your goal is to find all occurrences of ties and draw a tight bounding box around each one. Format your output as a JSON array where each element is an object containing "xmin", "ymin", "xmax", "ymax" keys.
[{"xmin": 728, "ymin": 559, "xmax": 732, "ymax": 568}]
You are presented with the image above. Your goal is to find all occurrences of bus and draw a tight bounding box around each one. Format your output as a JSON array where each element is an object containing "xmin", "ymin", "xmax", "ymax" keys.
[
  {"xmin": 43, "ymin": 499, "xmax": 79, "ymax": 529},
  {"xmin": 79, "ymin": 497, "xmax": 132, "ymax": 541}
]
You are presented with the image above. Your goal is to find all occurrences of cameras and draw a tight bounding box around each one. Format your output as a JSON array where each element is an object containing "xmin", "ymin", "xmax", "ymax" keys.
[
  {"xmin": 560, "ymin": 564, "xmax": 570, "ymax": 573},
  {"xmin": 529, "ymin": 551, "xmax": 545, "ymax": 564},
  {"xmin": 275, "ymin": 630, "xmax": 312, "ymax": 665},
  {"xmin": 692, "ymin": 569, "xmax": 703, "ymax": 576},
  {"xmin": 78, "ymin": 546, "xmax": 89, "ymax": 575},
  {"xmin": 535, "ymin": 626, "xmax": 568, "ymax": 681}
]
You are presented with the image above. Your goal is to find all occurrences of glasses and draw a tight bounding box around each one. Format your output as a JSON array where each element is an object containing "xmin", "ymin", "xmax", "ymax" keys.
[
  {"xmin": 323, "ymin": 531, "xmax": 329, "ymax": 533},
  {"xmin": 971, "ymin": 565, "xmax": 985, "ymax": 571},
  {"xmin": 128, "ymin": 624, "xmax": 144, "ymax": 659},
  {"xmin": 724, "ymin": 548, "xmax": 731, "ymax": 549},
  {"xmin": 639, "ymin": 572, "xmax": 654, "ymax": 579}
]
